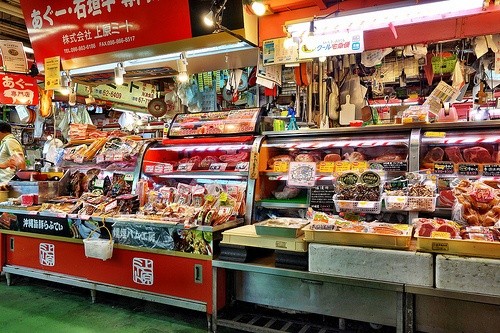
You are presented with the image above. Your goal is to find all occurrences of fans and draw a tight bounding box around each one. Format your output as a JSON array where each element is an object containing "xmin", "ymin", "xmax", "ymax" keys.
[{"xmin": 148, "ymin": 98, "xmax": 167, "ymax": 125}]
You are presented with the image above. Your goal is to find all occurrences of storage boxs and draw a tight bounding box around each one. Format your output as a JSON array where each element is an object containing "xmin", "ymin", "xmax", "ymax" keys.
[
  {"xmin": 332, "ymin": 186, "xmax": 383, "ymax": 214},
  {"xmin": 255, "ymin": 217, "xmax": 311, "ymax": 238},
  {"xmin": 301, "ymin": 224, "xmax": 414, "ymax": 250},
  {"xmin": 383, "ymin": 193, "xmax": 439, "ymax": 211},
  {"xmin": 413, "ymin": 227, "xmax": 500, "ymax": 259}
]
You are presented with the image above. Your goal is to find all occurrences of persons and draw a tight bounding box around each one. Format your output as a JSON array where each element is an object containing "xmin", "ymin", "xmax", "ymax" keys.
[{"xmin": 0, "ymin": 120, "xmax": 26, "ymax": 186}]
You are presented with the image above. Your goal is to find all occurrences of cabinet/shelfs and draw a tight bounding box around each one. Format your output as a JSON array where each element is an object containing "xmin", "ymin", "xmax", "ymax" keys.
[
  {"xmin": 245, "ymin": 123, "xmax": 500, "ymax": 227},
  {"xmin": 0, "ymin": 136, "xmax": 259, "ymax": 224}
]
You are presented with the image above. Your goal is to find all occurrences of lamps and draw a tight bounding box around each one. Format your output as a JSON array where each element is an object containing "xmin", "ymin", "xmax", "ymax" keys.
[
  {"xmin": 60, "ymin": 72, "xmax": 69, "ymax": 96},
  {"xmin": 114, "ymin": 63, "xmax": 125, "ymax": 86},
  {"xmin": 178, "ymin": 52, "xmax": 188, "ymax": 84},
  {"xmin": 204, "ymin": 0, "xmax": 229, "ymax": 33}
]
